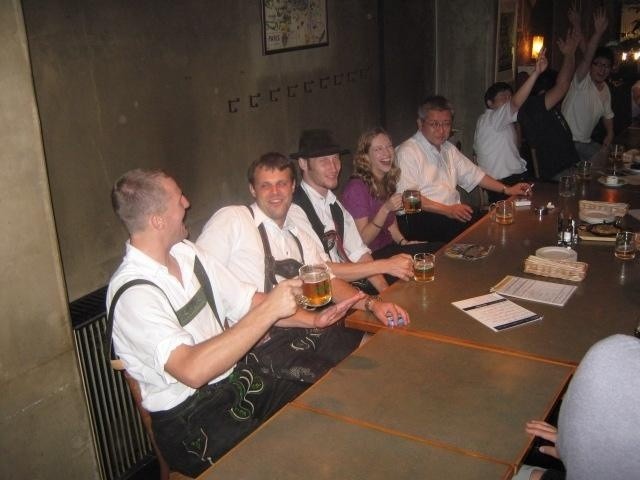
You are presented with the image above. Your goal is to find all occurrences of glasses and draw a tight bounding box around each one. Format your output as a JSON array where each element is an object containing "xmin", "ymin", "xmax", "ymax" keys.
[{"xmin": 532, "ymin": 31, "xmax": 545, "ymax": 60}]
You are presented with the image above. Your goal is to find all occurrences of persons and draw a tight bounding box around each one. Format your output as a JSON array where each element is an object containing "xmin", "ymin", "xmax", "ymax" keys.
[
  {"xmin": 105, "ymin": 167, "xmax": 366, "ymax": 478},
  {"xmin": 193, "ymin": 152, "xmax": 410, "ymax": 385},
  {"xmin": 508, "ymin": 419, "xmax": 568, "ymax": 479},
  {"xmin": 517, "ymin": 6, "xmax": 639, "ymax": 184},
  {"xmin": 393, "ymin": 94, "xmax": 532, "ymax": 245},
  {"xmin": 472, "ymin": 46, "xmax": 549, "ymax": 201},
  {"xmin": 286, "ymin": 128, "xmax": 414, "ymax": 294},
  {"xmin": 338, "ymin": 126, "xmax": 446, "ymax": 286}
]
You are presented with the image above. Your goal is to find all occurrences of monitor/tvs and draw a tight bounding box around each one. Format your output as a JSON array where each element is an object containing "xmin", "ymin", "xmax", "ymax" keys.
[
  {"xmin": 450, "ymin": 292, "xmax": 544, "ymax": 333},
  {"xmin": 491, "ymin": 274, "xmax": 578, "ymax": 308},
  {"xmin": 579, "ymin": 230, "xmax": 617, "ymax": 242}
]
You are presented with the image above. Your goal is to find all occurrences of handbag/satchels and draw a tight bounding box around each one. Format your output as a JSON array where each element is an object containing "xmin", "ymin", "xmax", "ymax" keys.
[
  {"xmin": 503, "ymin": 184, "xmax": 509, "ymax": 195},
  {"xmin": 371, "ymin": 221, "xmax": 382, "ymax": 229},
  {"xmin": 399, "ymin": 238, "xmax": 405, "ymax": 245},
  {"xmin": 364, "ymin": 295, "xmax": 378, "ymax": 310}
]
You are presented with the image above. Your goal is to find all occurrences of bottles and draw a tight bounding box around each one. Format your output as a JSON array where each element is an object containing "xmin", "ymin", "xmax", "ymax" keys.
[{"xmin": 287, "ymin": 128, "xmax": 351, "ymax": 160}]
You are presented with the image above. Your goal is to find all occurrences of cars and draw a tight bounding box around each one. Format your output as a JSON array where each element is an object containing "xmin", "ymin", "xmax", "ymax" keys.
[
  {"xmin": 578, "ymin": 183, "xmax": 591, "ymax": 199},
  {"xmin": 556, "ymin": 213, "xmax": 578, "ymax": 247},
  {"xmin": 559, "ymin": 175, "xmax": 578, "ymax": 198},
  {"xmin": 607, "ymin": 144, "xmax": 624, "ymax": 172},
  {"xmin": 577, "ymin": 160, "xmax": 592, "ymax": 180},
  {"xmin": 488, "ymin": 199, "xmax": 513, "ymax": 225},
  {"xmin": 414, "ymin": 253, "xmax": 435, "ymax": 283},
  {"xmin": 294, "ymin": 263, "xmax": 332, "ymax": 307},
  {"xmin": 614, "ymin": 231, "xmax": 636, "ymax": 261},
  {"xmin": 403, "ymin": 189, "xmax": 422, "ymax": 213}
]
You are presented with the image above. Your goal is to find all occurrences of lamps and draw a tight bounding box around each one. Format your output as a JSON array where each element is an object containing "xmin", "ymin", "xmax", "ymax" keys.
[{"xmin": 108, "ymin": 347, "xmax": 191, "ymax": 479}]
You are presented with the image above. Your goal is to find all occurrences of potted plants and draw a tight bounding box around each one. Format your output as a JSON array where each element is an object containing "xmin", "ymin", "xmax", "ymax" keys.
[
  {"xmin": 260, "ymin": 1, "xmax": 333, "ymax": 56},
  {"xmin": 493, "ymin": 1, "xmax": 521, "ymax": 91}
]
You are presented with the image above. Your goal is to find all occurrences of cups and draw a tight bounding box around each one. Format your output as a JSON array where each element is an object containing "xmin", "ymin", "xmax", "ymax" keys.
[{"xmin": 591, "ymin": 61, "xmax": 611, "ymax": 69}]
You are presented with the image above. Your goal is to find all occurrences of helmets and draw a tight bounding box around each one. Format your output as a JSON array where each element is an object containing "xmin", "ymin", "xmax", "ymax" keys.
[
  {"xmin": 536, "ymin": 246, "xmax": 578, "ymax": 263},
  {"xmin": 624, "ymin": 163, "xmax": 640, "ymax": 173},
  {"xmin": 586, "ymin": 223, "xmax": 622, "ymax": 236},
  {"xmin": 578, "ymin": 208, "xmax": 616, "ymax": 225},
  {"xmin": 598, "ymin": 176, "xmax": 628, "ymax": 187}
]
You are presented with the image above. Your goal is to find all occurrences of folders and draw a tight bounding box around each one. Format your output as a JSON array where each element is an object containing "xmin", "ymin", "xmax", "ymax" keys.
[{"xmin": 68, "ymin": 275, "xmax": 158, "ymax": 480}]
